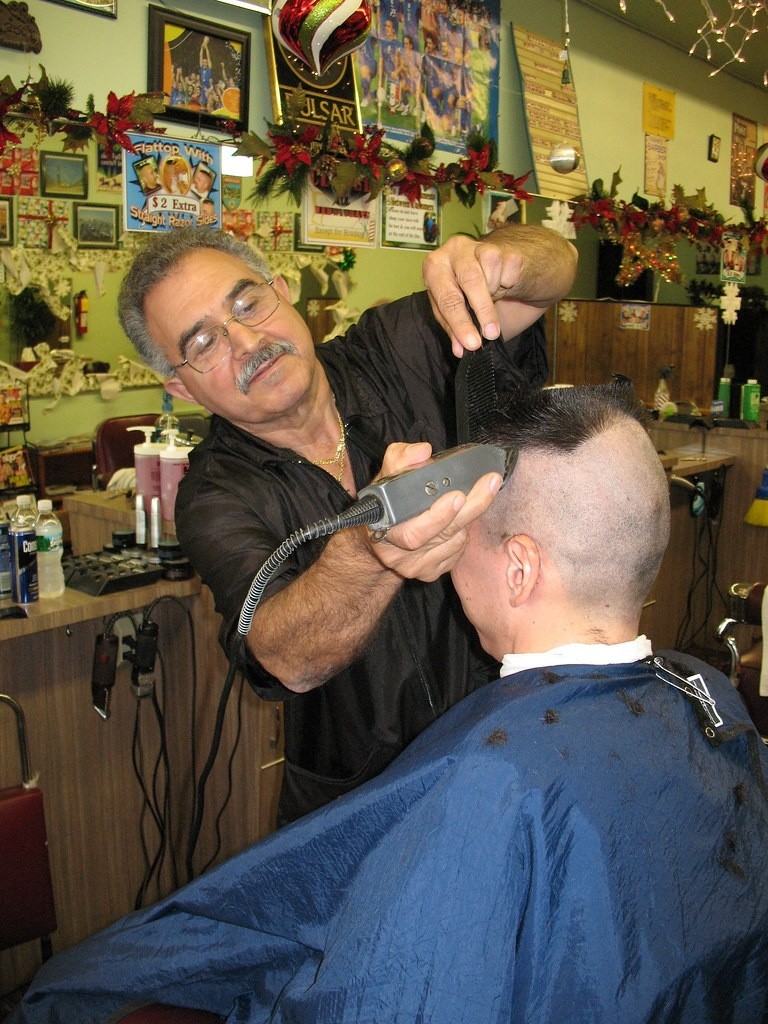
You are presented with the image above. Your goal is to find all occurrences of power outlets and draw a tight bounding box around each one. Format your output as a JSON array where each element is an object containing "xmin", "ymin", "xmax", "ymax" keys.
[{"xmin": 113, "ymin": 613, "xmax": 143, "ymax": 667}]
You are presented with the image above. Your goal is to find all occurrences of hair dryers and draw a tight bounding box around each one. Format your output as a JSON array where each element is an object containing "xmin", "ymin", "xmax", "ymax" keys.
[{"xmin": 657, "ymin": 449, "xmax": 698, "ymax": 497}]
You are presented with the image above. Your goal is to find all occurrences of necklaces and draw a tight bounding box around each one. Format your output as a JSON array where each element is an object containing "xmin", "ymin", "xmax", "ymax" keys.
[{"xmin": 311, "ymin": 394, "xmax": 346, "ymax": 482}]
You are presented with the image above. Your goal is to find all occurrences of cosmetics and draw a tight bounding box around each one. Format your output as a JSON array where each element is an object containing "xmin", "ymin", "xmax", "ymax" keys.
[
  {"xmin": 126, "ymin": 426, "xmax": 166, "ymax": 516},
  {"xmin": 159, "ymin": 429, "xmax": 194, "ymax": 520}
]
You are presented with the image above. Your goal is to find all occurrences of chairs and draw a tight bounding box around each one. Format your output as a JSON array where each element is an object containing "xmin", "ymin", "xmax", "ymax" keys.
[
  {"xmin": 89, "ymin": 413, "xmax": 163, "ymax": 492},
  {"xmin": 174, "ymin": 414, "xmax": 208, "ymax": 440},
  {"xmin": 713, "ymin": 581, "xmax": 768, "ymax": 722},
  {"xmin": 0, "ymin": 695, "xmax": 222, "ymax": 1024}
]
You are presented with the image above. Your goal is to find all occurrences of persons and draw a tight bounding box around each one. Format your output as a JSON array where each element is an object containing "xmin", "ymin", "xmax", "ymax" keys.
[
  {"xmin": 140, "ymin": 162, "xmax": 162, "ymax": 193},
  {"xmin": 190, "ymin": 170, "xmax": 211, "ymax": 196},
  {"xmin": 359, "ymin": 0, "xmax": 491, "ymax": 142},
  {"xmin": 117, "ymin": 224, "xmax": 578, "ymax": 830},
  {"xmin": 11, "ymin": 389, "xmax": 768, "ymax": 1024},
  {"xmin": 171, "ymin": 45, "xmax": 236, "ymax": 112}
]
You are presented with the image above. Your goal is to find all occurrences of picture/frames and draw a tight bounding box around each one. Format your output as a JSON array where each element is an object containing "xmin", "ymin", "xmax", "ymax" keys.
[
  {"xmin": 38, "ymin": 0, "xmax": 118, "ymax": 20},
  {"xmin": 72, "ymin": 201, "xmax": 121, "ymax": 251},
  {"xmin": 39, "ymin": 150, "xmax": 89, "ymax": 200},
  {"xmin": 146, "ymin": 3, "xmax": 252, "ymax": 135},
  {"xmin": 294, "ymin": 213, "xmax": 326, "ymax": 254},
  {"xmin": 707, "ymin": 134, "xmax": 721, "ymax": 163},
  {"xmin": 0, "ymin": 195, "xmax": 14, "ymax": 247}
]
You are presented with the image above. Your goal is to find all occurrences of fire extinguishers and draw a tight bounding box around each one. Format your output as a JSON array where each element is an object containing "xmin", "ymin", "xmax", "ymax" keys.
[{"xmin": 74, "ymin": 290, "xmax": 88, "ymax": 333}]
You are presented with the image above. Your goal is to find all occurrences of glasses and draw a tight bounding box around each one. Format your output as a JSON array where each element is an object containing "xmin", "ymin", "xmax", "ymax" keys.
[{"xmin": 168, "ymin": 277, "xmax": 281, "ymax": 373}]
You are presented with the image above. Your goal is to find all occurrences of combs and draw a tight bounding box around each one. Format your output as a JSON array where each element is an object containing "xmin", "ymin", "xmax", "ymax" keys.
[{"xmin": 455, "ymin": 308, "xmax": 499, "ymax": 445}]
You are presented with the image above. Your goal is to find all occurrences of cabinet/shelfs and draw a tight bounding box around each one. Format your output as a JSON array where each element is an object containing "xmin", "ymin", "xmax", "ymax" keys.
[
  {"xmin": 63, "ymin": 491, "xmax": 179, "ymax": 556},
  {"xmin": 28, "ymin": 441, "xmax": 95, "ymax": 510},
  {"xmin": 0, "ymin": 423, "xmax": 40, "ymax": 501}
]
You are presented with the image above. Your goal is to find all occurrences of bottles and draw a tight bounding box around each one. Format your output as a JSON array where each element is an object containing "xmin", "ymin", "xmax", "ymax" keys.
[
  {"xmin": 8, "ymin": 495, "xmax": 39, "ymax": 603},
  {"xmin": 0, "ymin": 508, "xmax": 13, "ymax": 600},
  {"xmin": 92, "ymin": 465, "xmax": 99, "ymax": 493},
  {"xmin": 740, "ymin": 379, "xmax": 760, "ymax": 422},
  {"xmin": 9, "ymin": 522, "xmax": 38, "ymax": 604},
  {"xmin": 36, "ymin": 499, "xmax": 65, "ymax": 598},
  {"xmin": 718, "ymin": 378, "xmax": 731, "ymax": 417}
]
